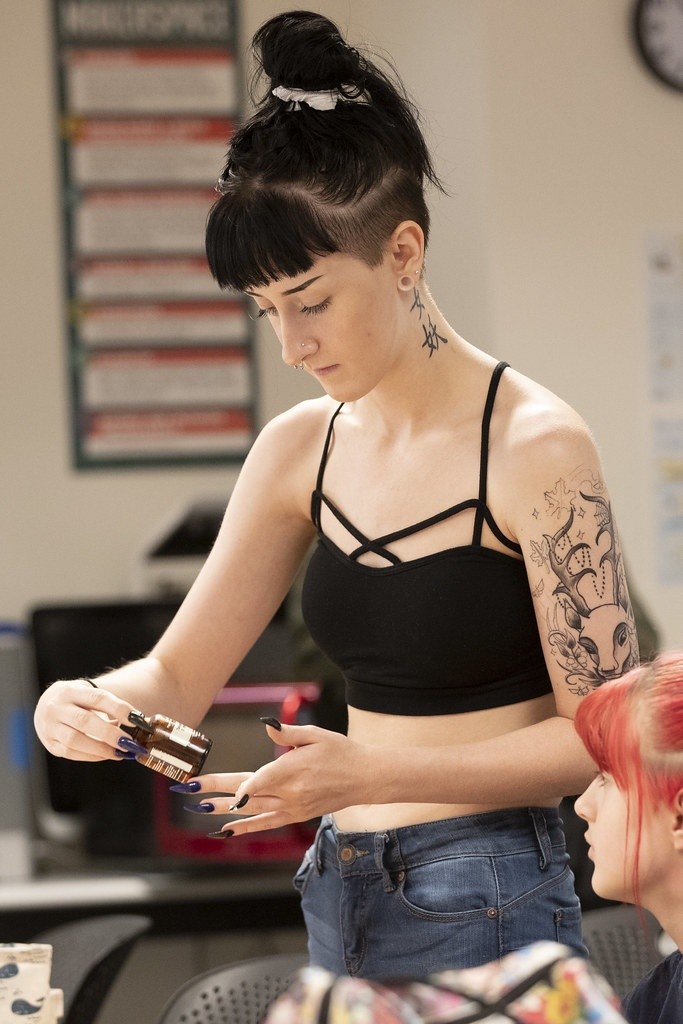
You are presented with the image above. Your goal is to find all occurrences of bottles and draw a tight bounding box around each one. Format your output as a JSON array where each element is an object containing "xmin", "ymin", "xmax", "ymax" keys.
[{"xmin": 117, "ymin": 713, "xmax": 214, "ymax": 784}]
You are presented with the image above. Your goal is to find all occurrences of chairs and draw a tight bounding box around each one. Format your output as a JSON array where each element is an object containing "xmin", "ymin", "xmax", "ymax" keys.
[
  {"xmin": 157, "ymin": 945, "xmax": 311, "ymax": 1024},
  {"xmin": 30, "ymin": 914, "xmax": 153, "ymax": 1024},
  {"xmin": 28, "ymin": 593, "xmax": 289, "ymax": 856},
  {"xmin": 577, "ymin": 904, "xmax": 666, "ymax": 1008}
]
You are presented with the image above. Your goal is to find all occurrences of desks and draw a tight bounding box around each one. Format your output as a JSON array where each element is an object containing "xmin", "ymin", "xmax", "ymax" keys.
[{"xmin": 0, "ymin": 862, "xmax": 320, "ymax": 943}]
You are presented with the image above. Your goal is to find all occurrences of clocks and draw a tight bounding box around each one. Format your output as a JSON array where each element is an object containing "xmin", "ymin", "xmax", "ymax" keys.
[{"xmin": 636, "ymin": 0, "xmax": 683, "ymax": 95}]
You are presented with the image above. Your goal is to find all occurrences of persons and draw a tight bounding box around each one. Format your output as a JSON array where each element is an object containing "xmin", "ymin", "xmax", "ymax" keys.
[
  {"xmin": 33, "ymin": 11, "xmax": 642, "ymax": 986},
  {"xmin": 574, "ymin": 650, "xmax": 682, "ymax": 1024}
]
